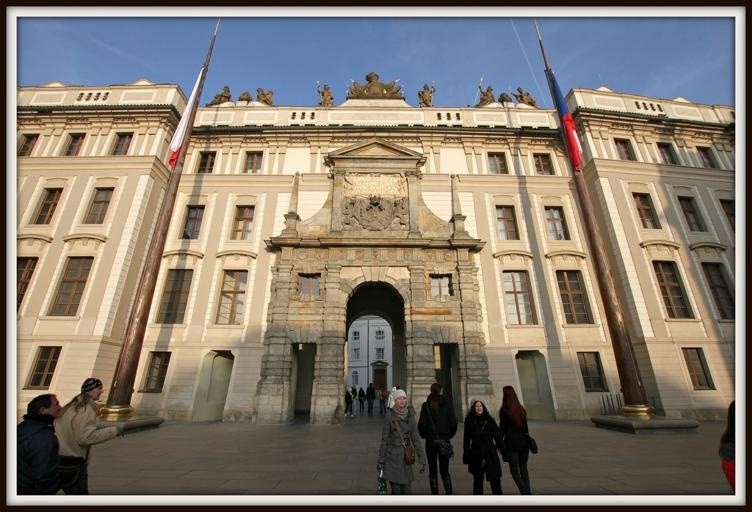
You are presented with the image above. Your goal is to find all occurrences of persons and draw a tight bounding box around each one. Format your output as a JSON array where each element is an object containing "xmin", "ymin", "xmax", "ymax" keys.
[
  {"xmin": 17, "ymin": 393, "xmax": 70, "ymax": 495},
  {"xmin": 716, "ymin": 399, "xmax": 734, "ymax": 492},
  {"xmin": 418, "ymin": 83, "xmax": 436, "ymax": 107},
  {"xmin": 461, "ymin": 395, "xmax": 509, "ymax": 494},
  {"xmin": 511, "ymin": 87, "xmax": 536, "ymax": 107},
  {"xmin": 317, "ymin": 84, "xmax": 335, "ymax": 107},
  {"xmin": 53, "ymin": 377, "xmax": 124, "ymax": 495},
  {"xmin": 344, "ymin": 381, "xmax": 403, "ymax": 417},
  {"xmin": 478, "ymin": 85, "xmax": 496, "ymax": 107},
  {"xmin": 206, "ymin": 86, "xmax": 231, "ymax": 106},
  {"xmin": 376, "ymin": 389, "xmax": 426, "ymax": 494},
  {"xmin": 496, "ymin": 385, "xmax": 537, "ymax": 494},
  {"xmin": 418, "ymin": 382, "xmax": 458, "ymax": 493}
]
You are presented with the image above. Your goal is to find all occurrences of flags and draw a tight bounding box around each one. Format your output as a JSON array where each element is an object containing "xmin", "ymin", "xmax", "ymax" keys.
[
  {"xmin": 165, "ymin": 61, "xmax": 207, "ymax": 170},
  {"xmin": 544, "ymin": 63, "xmax": 583, "ymax": 172}
]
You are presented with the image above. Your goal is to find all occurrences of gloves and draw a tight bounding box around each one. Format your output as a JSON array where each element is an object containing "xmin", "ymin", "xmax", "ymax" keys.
[
  {"xmin": 377, "ymin": 463, "xmax": 385, "ymax": 472},
  {"xmin": 419, "ymin": 464, "xmax": 425, "ymax": 474}
]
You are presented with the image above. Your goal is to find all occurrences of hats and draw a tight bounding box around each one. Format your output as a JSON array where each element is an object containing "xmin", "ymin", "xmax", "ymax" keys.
[
  {"xmin": 392, "ymin": 389, "xmax": 407, "ymax": 400},
  {"xmin": 470, "ymin": 396, "xmax": 487, "ymax": 408},
  {"xmin": 81, "ymin": 378, "xmax": 103, "ymax": 394}
]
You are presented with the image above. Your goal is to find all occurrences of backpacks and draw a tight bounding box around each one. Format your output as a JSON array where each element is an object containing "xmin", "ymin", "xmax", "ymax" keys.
[{"xmin": 380, "ymin": 388, "xmax": 387, "ymax": 398}]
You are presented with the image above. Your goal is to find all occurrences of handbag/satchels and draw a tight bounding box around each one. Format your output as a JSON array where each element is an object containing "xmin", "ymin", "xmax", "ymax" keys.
[
  {"xmin": 376, "ymin": 470, "xmax": 387, "ymax": 494},
  {"xmin": 529, "ymin": 436, "xmax": 537, "ymax": 455},
  {"xmin": 362, "ymin": 397, "xmax": 365, "ymax": 402},
  {"xmin": 57, "ymin": 462, "xmax": 86, "ymax": 488},
  {"xmin": 437, "ymin": 438, "xmax": 454, "ymax": 458},
  {"xmin": 406, "ymin": 445, "xmax": 416, "ymax": 464}
]
